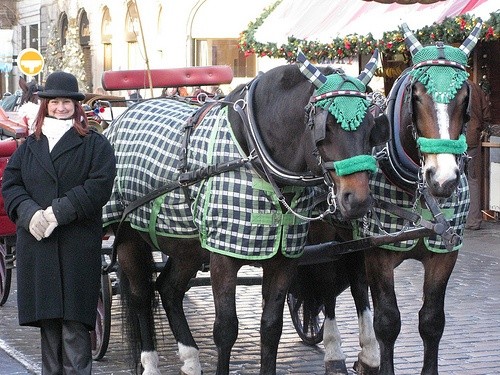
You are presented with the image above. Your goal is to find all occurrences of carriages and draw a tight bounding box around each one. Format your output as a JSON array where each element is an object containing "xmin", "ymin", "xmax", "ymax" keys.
[{"xmin": 0, "ymin": 19, "xmax": 486, "ymax": 375}]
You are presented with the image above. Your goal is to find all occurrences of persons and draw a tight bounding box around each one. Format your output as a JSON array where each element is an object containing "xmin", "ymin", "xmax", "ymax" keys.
[
  {"xmin": 2, "ymin": 71, "xmax": 116, "ymax": 375},
  {"xmin": 463, "ymin": 80, "xmax": 491, "ymax": 231}
]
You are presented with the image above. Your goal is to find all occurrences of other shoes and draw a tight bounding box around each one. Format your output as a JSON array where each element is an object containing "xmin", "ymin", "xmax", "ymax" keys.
[{"xmin": 464, "ymin": 224, "xmax": 480, "ymax": 230}]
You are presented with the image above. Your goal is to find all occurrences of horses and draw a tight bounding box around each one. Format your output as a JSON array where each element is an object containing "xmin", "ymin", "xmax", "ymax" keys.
[
  {"xmin": 1, "ymin": 75, "xmax": 46, "ymax": 139},
  {"xmin": 93, "ymin": 46, "xmax": 382, "ymax": 375},
  {"xmin": 315, "ymin": 22, "xmax": 483, "ymax": 375}
]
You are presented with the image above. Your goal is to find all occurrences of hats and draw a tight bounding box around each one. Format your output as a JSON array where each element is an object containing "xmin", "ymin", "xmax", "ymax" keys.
[{"xmin": 36, "ymin": 71, "xmax": 85, "ymax": 102}]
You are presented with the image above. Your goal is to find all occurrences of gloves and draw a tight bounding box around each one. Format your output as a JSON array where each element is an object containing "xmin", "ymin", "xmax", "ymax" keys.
[
  {"xmin": 43, "ymin": 206, "xmax": 59, "ymax": 237},
  {"xmin": 28, "ymin": 209, "xmax": 49, "ymax": 241}
]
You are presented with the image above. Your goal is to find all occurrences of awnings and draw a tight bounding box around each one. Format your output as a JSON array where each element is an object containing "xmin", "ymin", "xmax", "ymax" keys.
[{"xmin": 239, "ymin": 1, "xmax": 500, "ymax": 63}]
